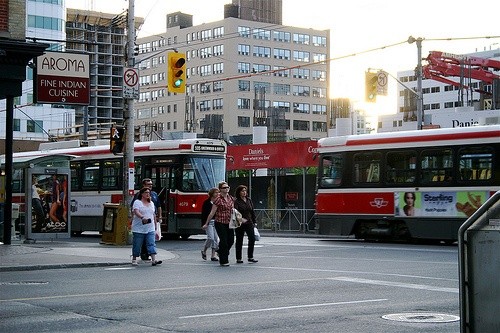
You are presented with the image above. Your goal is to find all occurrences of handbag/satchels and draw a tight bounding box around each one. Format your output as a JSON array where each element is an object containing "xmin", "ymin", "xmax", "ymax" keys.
[
  {"xmin": 155, "ymin": 222, "xmax": 161, "ymax": 242},
  {"xmin": 229, "ymin": 209, "xmax": 242, "ymax": 229},
  {"xmin": 254, "ymin": 227, "xmax": 260, "ymax": 241}
]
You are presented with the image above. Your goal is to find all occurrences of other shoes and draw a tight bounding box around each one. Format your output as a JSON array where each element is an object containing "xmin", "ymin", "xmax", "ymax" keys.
[
  {"xmin": 152, "ymin": 259, "xmax": 162, "ymax": 266},
  {"xmin": 248, "ymin": 259, "xmax": 258, "ymax": 262},
  {"xmin": 201, "ymin": 251, "xmax": 207, "ymax": 260},
  {"xmin": 132, "ymin": 259, "xmax": 137, "ymax": 265},
  {"xmin": 211, "ymin": 257, "xmax": 218, "ymax": 261},
  {"xmin": 237, "ymin": 259, "xmax": 244, "ymax": 263}
]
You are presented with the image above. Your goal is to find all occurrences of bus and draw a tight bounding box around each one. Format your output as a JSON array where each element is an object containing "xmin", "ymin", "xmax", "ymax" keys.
[
  {"xmin": 0, "ymin": 105, "xmax": 228, "ymax": 241},
  {"xmin": 311, "ymin": 125, "xmax": 500, "ymax": 245}
]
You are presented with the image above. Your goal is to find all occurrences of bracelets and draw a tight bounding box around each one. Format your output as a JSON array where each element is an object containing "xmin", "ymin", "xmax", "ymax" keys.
[{"xmin": 141, "ymin": 216, "xmax": 144, "ymax": 219}]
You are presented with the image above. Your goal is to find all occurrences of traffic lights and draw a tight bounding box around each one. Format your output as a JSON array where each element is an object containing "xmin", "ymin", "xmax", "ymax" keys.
[
  {"xmin": 110, "ymin": 141, "xmax": 124, "ymax": 153},
  {"xmin": 365, "ymin": 71, "xmax": 379, "ymax": 103},
  {"xmin": 166, "ymin": 51, "xmax": 187, "ymax": 94},
  {"xmin": 110, "ymin": 126, "xmax": 125, "ymax": 141}
]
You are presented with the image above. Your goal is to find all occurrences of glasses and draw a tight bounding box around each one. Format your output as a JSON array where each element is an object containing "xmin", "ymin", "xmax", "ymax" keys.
[
  {"xmin": 147, "ymin": 184, "xmax": 153, "ymax": 185},
  {"xmin": 223, "ymin": 186, "xmax": 229, "ymax": 189}
]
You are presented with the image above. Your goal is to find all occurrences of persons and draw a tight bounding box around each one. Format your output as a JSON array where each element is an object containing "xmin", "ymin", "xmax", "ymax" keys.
[
  {"xmin": 132, "ymin": 188, "xmax": 162, "ymax": 266},
  {"xmin": 203, "ymin": 182, "xmax": 234, "ymax": 266},
  {"xmin": 130, "ymin": 178, "xmax": 161, "ymax": 260},
  {"xmin": 399, "ymin": 192, "xmax": 421, "ymax": 216},
  {"xmin": 32, "ymin": 174, "xmax": 67, "ymax": 231},
  {"xmin": 201, "ymin": 187, "xmax": 220, "ymax": 261},
  {"xmin": 234, "ymin": 185, "xmax": 258, "ymax": 263}
]
